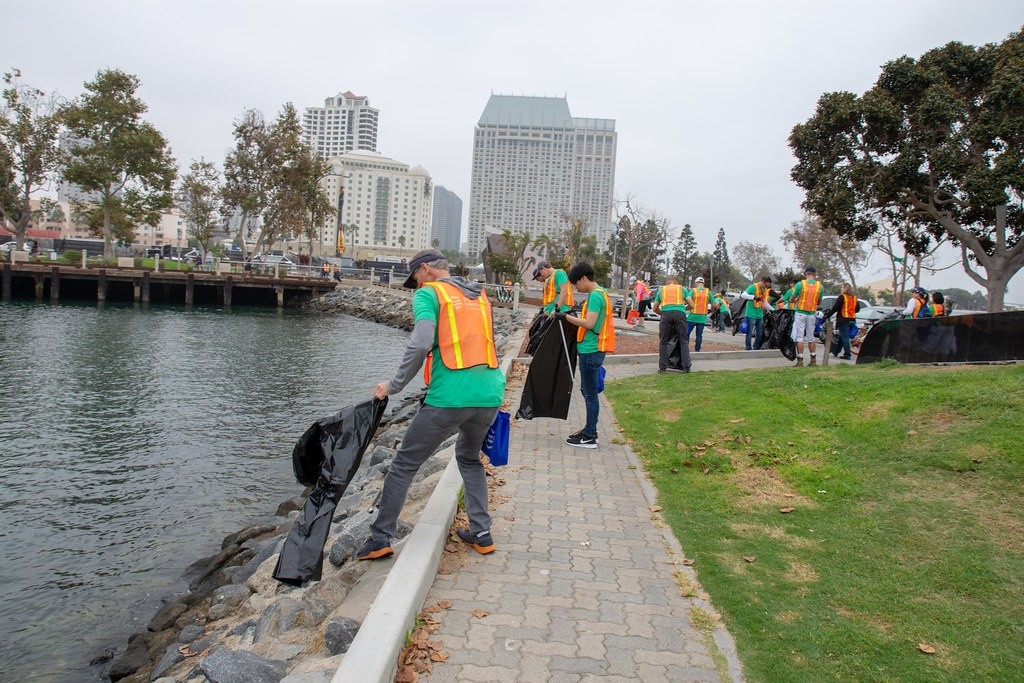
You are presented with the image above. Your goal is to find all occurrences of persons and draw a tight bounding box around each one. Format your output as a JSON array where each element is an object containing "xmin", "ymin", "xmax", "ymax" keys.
[
  {"xmin": 686, "ymin": 277, "xmax": 715, "ymax": 349},
  {"xmin": 740, "ymin": 276, "xmax": 772, "ymax": 350},
  {"xmin": 504, "ymin": 277, "xmax": 512, "ymax": 298},
  {"xmin": 532, "ymin": 269, "xmax": 550, "ymax": 312},
  {"xmin": 334, "ymin": 262, "xmax": 339, "ymax": 279},
  {"xmin": 356, "ymin": 250, "xmax": 506, "ymax": 558},
  {"xmin": 820, "ymin": 283, "xmax": 860, "ymax": 359},
  {"xmin": 789, "ymin": 267, "xmax": 824, "ymax": 366},
  {"xmin": 629, "ymin": 276, "xmax": 651, "ymax": 328},
  {"xmin": 715, "ymin": 298, "xmax": 729, "ymax": 333},
  {"xmin": 766, "ymin": 279, "xmax": 801, "ymax": 309},
  {"xmin": 323, "ymin": 262, "xmax": 330, "ymax": 279},
  {"xmin": 902, "ymin": 287, "xmax": 928, "ymax": 319},
  {"xmin": 535, "ymin": 261, "xmax": 575, "ymax": 318},
  {"xmin": 653, "ymin": 275, "xmax": 692, "ymax": 373},
  {"xmin": 928, "ymin": 291, "xmax": 946, "ymax": 317},
  {"xmin": 549, "ymin": 261, "xmax": 615, "ymax": 448},
  {"xmin": 711, "ymin": 290, "xmax": 725, "ymax": 331}
]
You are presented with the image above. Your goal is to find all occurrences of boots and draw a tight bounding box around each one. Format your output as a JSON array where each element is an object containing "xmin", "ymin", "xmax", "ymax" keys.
[
  {"xmin": 794, "ymin": 358, "xmax": 804, "ymax": 367},
  {"xmin": 808, "ymin": 356, "xmax": 816, "ymax": 366}
]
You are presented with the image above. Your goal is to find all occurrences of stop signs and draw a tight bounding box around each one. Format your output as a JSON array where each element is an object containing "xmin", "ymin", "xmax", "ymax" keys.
[{"xmin": 403, "ymin": 258, "xmax": 406, "ymax": 263}]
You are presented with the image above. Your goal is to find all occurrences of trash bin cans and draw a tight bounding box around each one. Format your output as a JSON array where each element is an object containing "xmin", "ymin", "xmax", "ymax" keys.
[
  {"xmin": 380, "ymin": 267, "xmax": 389, "ymax": 283},
  {"xmin": 740, "ymin": 317, "xmax": 748, "ymax": 333}
]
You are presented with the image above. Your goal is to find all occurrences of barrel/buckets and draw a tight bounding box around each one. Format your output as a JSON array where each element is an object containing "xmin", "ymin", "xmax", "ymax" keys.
[{"xmin": 627, "ymin": 310, "xmax": 639, "ymax": 326}]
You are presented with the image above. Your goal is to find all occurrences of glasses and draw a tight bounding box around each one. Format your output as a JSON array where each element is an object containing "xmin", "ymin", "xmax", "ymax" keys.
[{"xmin": 764, "ymin": 282, "xmax": 768, "ymax": 288}]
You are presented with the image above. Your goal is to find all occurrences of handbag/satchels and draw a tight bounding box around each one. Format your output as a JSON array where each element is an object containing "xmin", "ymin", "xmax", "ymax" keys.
[
  {"xmin": 597, "ymin": 365, "xmax": 607, "ymax": 393},
  {"xmin": 480, "ymin": 409, "xmax": 511, "ymax": 466},
  {"xmin": 627, "ymin": 310, "xmax": 639, "ymax": 325}
]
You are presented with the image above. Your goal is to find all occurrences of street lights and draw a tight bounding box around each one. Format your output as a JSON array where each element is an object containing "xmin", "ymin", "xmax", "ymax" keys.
[
  {"xmin": 308, "ymin": 173, "xmax": 350, "ymax": 280},
  {"xmin": 611, "ymin": 228, "xmax": 626, "ymax": 288}
]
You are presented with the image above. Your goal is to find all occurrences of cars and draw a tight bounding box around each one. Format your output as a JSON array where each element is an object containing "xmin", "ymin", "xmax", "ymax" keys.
[
  {"xmin": 325, "ymin": 255, "xmax": 358, "ymax": 274},
  {"xmin": 251, "ymin": 254, "xmax": 293, "ymax": 275},
  {"xmin": 181, "ymin": 248, "xmax": 229, "ymax": 267},
  {"xmin": 0, "ymin": 241, "xmax": 31, "ymax": 254},
  {"xmin": 804, "ymin": 296, "xmax": 908, "ymax": 356},
  {"xmin": 612, "ymin": 283, "xmax": 661, "ymax": 320}
]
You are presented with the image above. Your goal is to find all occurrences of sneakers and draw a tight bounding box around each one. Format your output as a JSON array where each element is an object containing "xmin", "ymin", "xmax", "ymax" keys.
[
  {"xmin": 356, "ymin": 537, "xmax": 394, "ymax": 560},
  {"xmin": 566, "ymin": 435, "xmax": 597, "ymax": 449},
  {"xmin": 569, "ymin": 432, "xmax": 598, "ymax": 443},
  {"xmin": 457, "ymin": 528, "xmax": 495, "ymax": 555}
]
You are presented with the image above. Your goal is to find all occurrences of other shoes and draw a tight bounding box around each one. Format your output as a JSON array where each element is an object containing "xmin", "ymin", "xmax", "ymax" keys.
[
  {"xmin": 840, "ymin": 355, "xmax": 850, "ymax": 359},
  {"xmin": 833, "ymin": 350, "xmax": 838, "ymax": 357}
]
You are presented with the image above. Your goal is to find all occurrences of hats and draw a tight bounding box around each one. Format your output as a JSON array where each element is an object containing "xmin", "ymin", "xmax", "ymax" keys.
[
  {"xmin": 695, "ymin": 277, "xmax": 704, "ymax": 283},
  {"xmin": 532, "ymin": 269, "xmax": 539, "ymax": 280},
  {"xmin": 804, "ymin": 267, "xmax": 816, "ymax": 274},
  {"xmin": 909, "ymin": 287, "xmax": 924, "ymax": 295},
  {"xmin": 403, "ymin": 249, "xmax": 446, "ymax": 289},
  {"xmin": 629, "ymin": 276, "xmax": 636, "ymax": 285},
  {"xmin": 534, "ymin": 260, "xmax": 548, "ymax": 276}
]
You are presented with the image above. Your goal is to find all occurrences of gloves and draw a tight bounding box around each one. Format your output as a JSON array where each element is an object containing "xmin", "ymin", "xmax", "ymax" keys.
[{"xmin": 754, "ymin": 296, "xmax": 761, "ymax": 302}]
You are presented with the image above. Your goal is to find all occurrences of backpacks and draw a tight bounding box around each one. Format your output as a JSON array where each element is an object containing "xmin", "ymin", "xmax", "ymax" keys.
[{"xmin": 917, "ymin": 298, "xmax": 932, "ymax": 318}]
